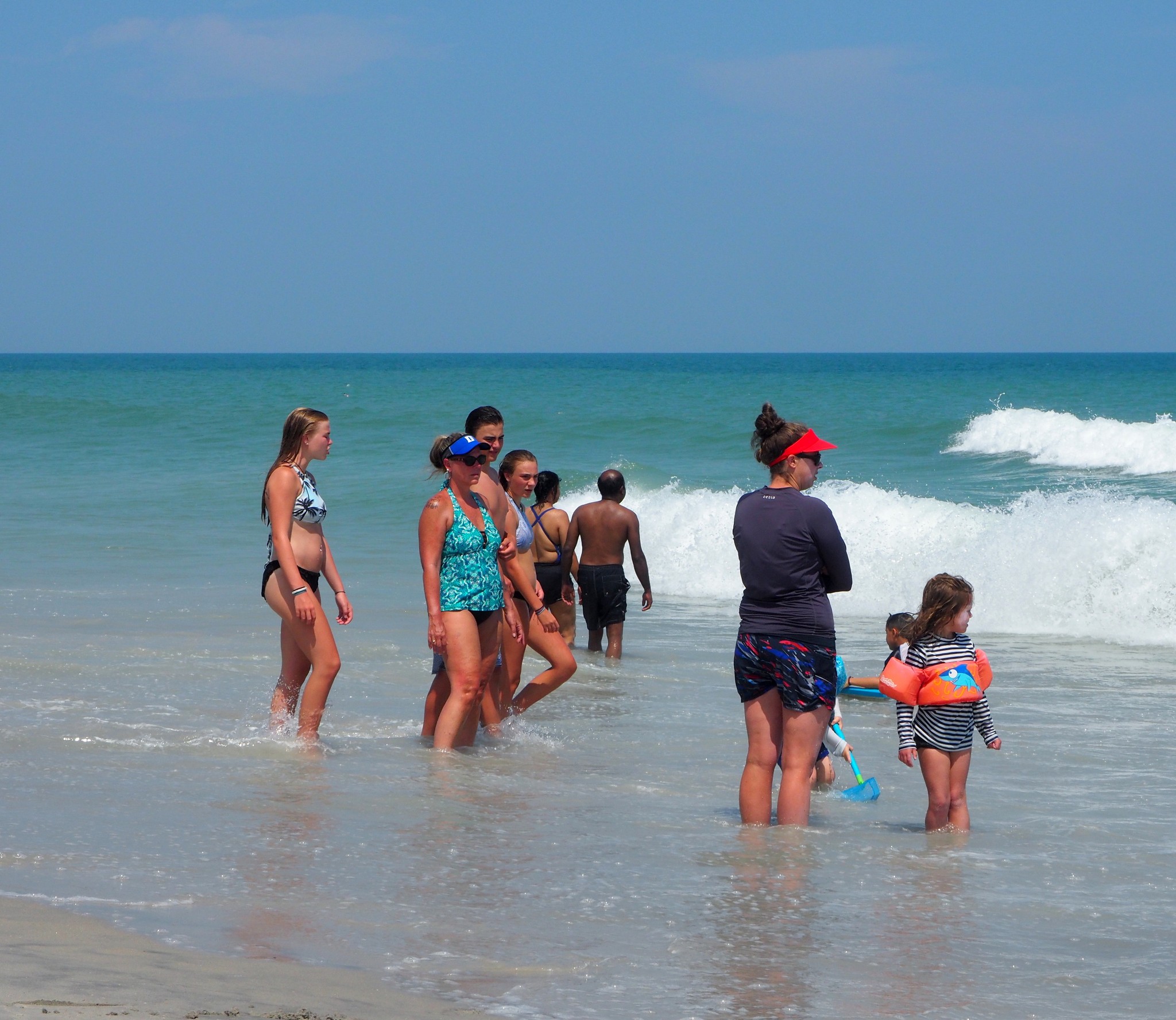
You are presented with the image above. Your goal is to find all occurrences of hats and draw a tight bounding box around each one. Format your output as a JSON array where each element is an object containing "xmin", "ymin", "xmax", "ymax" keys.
[
  {"xmin": 440, "ymin": 434, "xmax": 491, "ymax": 463},
  {"xmin": 769, "ymin": 428, "xmax": 838, "ymax": 467}
]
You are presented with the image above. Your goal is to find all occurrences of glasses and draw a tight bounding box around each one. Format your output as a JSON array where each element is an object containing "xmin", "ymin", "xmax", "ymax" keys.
[
  {"xmin": 448, "ymin": 454, "xmax": 486, "ymax": 466},
  {"xmin": 797, "ymin": 453, "xmax": 821, "ymax": 466}
]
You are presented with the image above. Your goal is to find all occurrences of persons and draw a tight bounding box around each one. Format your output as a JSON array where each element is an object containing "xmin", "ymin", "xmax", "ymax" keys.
[
  {"xmin": 418, "ymin": 434, "xmax": 523, "ymax": 747},
  {"xmin": 524, "ymin": 471, "xmax": 584, "ymax": 644},
  {"xmin": 421, "ymin": 406, "xmax": 578, "ymax": 738},
  {"xmin": 896, "ymin": 573, "xmax": 1001, "ymax": 831},
  {"xmin": 732, "ymin": 402, "xmax": 852, "ymax": 828},
  {"xmin": 843, "ymin": 613, "xmax": 916, "ymax": 688},
  {"xmin": 261, "ymin": 408, "xmax": 354, "ymax": 743},
  {"xmin": 561, "ymin": 469, "xmax": 653, "ymax": 659},
  {"xmin": 777, "ymin": 655, "xmax": 853, "ymax": 789}
]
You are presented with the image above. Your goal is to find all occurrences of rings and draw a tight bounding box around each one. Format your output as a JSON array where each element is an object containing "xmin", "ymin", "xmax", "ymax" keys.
[{"xmin": 432, "ymin": 640, "xmax": 436, "ymax": 643}]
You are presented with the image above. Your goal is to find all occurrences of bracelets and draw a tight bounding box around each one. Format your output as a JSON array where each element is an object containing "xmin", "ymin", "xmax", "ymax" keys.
[
  {"xmin": 848, "ymin": 675, "xmax": 853, "ymax": 686},
  {"xmin": 291, "ymin": 586, "xmax": 308, "ymax": 595},
  {"xmin": 335, "ymin": 591, "xmax": 345, "ymax": 595},
  {"xmin": 533, "ymin": 605, "xmax": 545, "ymax": 625}
]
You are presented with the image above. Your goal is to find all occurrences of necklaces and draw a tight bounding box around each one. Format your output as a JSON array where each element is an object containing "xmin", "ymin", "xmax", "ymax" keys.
[{"xmin": 287, "ymin": 457, "xmax": 307, "ymax": 476}]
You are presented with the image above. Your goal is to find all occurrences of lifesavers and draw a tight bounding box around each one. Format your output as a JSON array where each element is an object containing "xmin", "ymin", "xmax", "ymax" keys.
[{"xmin": 877, "ymin": 648, "xmax": 993, "ymax": 707}]
